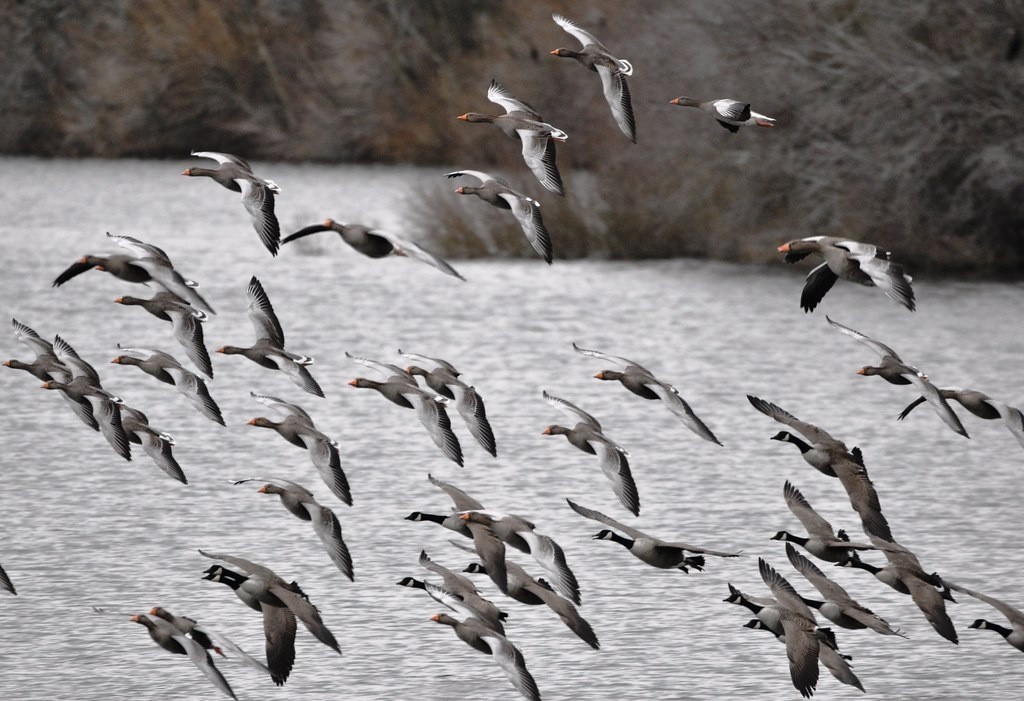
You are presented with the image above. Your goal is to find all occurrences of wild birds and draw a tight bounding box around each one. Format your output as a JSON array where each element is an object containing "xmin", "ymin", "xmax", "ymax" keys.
[
  {"xmin": 423, "ymin": 580, "xmax": 541, "ymax": 701},
  {"xmin": 896, "ymin": 388, "xmax": 1003, "ymax": 421},
  {"xmin": 1003, "ymin": 404, "xmax": 1024, "ymax": 450},
  {"xmin": 0, "ymin": 231, "xmax": 961, "ymax": 656},
  {"xmin": 740, "ymin": 593, "xmax": 866, "ymax": 693},
  {"xmin": 723, "ymin": 556, "xmax": 839, "ymax": 699},
  {"xmin": 148, "ymin": 607, "xmax": 279, "ymax": 678},
  {"xmin": 200, "ymin": 572, "xmax": 297, "ymax": 686},
  {"xmin": 180, "ymin": 149, "xmax": 282, "ymax": 258},
  {"xmin": 825, "ymin": 314, "xmax": 972, "ymax": 440},
  {"xmin": 119, "ymin": 611, "xmax": 238, "ymax": 701},
  {"xmin": 443, "ymin": 170, "xmax": 553, "ymax": 267},
  {"xmin": 669, "ymin": 96, "xmax": 777, "ymax": 134},
  {"xmin": 549, "ymin": 10, "xmax": 638, "ymax": 146},
  {"xmin": 456, "ymin": 78, "xmax": 569, "ymax": 197},
  {"xmin": 940, "ymin": 579, "xmax": 1024, "ymax": 653},
  {"xmin": 279, "ymin": 218, "xmax": 466, "ymax": 283}
]
